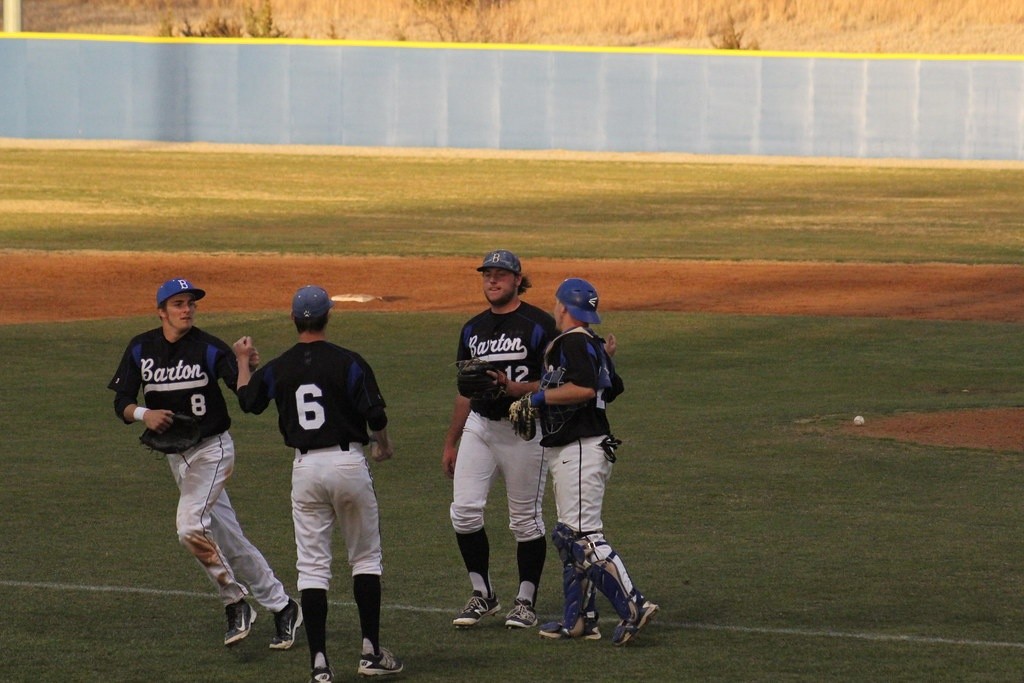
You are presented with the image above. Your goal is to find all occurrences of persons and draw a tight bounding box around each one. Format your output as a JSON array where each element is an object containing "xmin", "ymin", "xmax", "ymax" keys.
[
  {"xmin": 106, "ymin": 279, "xmax": 303, "ymax": 650},
  {"xmin": 442, "ymin": 249, "xmax": 563, "ymax": 629},
  {"xmin": 232, "ymin": 285, "xmax": 403, "ymax": 683},
  {"xmin": 508, "ymin": 278, "xmax": 659, "ymax": 646}
]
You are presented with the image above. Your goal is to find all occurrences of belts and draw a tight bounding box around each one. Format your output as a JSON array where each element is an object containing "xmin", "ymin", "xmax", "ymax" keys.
[{"xmin": 300, "ymin": 442, "xmax": 350, "ymax": 455}]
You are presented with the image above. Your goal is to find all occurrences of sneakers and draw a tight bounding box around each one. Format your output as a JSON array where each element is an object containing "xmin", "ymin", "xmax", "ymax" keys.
[
  {"xmin": 612, "ymin": 594, "xmax": 660, "ymax": 646},
  {"xmin": 224, "ymin": 599, "xmax": 257, "ymax": 645},
  {"xmin": 358, "ymin": 646, "xmax": 403, "ymax": 675},
  {"xmin": 308, "ymin": 666, "xmax": 336, "ymax": 683},
  {"xmin": 505, "ymin": 598, "xmax": 537, "ymax": 628},
  {"xmin": 452, "ymin": 590, "xmax": 501, "ymax": 626},
  {"xmin": 538, "ymin": 612, "xmax": 601, "ymax": 640},
  {"xmin": 269, "ymin": 598, "xmax": 303, "ymax": 648}
]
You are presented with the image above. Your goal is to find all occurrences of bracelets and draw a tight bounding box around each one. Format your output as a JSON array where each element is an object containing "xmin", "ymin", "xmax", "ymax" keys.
[
  {"xmin": 133, "ymin": 407, "xmax": 149, "ymax": 421},
  {"xmin": 531, "ymin": 391, "xmax": 545, "ymax": 408}
]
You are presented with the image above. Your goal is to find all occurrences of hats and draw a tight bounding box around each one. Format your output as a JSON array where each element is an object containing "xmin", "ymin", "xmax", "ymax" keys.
[
  {"xmin": 156, "ymin": 279, "xmax": 206, "ymax": 305},
  {"xmin": 477, "ymin": 249, "xmax": 521, "ymax": 275},
  {"xmin": 292, "ymin": 286, "xmax": 335, "ymax": 318}
]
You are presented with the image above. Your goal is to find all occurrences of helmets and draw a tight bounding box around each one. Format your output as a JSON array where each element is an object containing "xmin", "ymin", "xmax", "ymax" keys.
[{"xmin": 555, "ymin": 278, "xmax": 601, "ymax": 324}]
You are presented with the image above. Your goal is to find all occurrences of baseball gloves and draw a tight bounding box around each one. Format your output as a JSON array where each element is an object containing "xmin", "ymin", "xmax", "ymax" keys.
[
  {"xmin": 457, "ymin": 358, "xmax": 507, "ymax": 401},
  {"xmin": 510, "ymin": 392, "xmax": 538, "ymax": 441},
  {"xmin": 139, "ymin": 414, "xmax": 202, "ymax": 454}
]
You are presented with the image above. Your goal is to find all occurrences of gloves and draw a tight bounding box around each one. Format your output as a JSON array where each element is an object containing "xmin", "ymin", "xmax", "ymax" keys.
[{"xmin": 600, "ymin": 435, "xmax": 622, "ymax": 463}]
